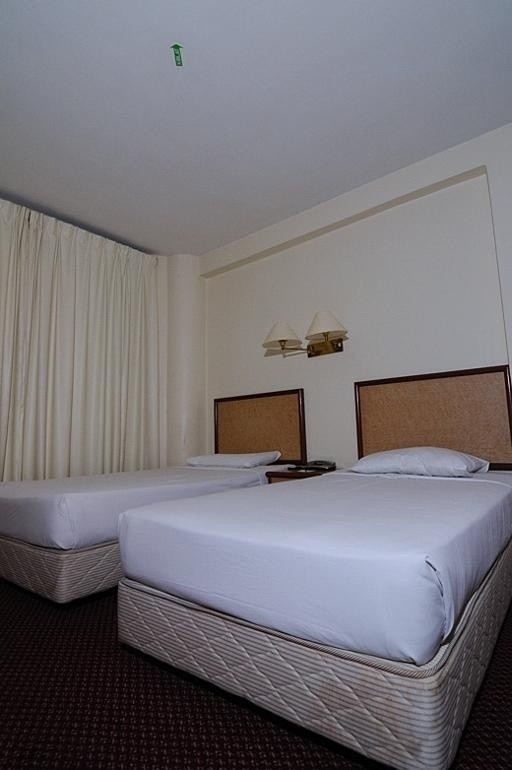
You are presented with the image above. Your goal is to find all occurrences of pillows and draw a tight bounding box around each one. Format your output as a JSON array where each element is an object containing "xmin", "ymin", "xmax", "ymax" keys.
[
  {"xmin": 349, "ymin": 444, "xmax": 491, "ymax": 478},
  {"xmin": 185, "ymin": 450, "xmax": 282, "ymax": 469}
]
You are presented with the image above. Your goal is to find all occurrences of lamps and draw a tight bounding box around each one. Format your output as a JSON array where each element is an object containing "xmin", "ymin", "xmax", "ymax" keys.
[{"xmin": 264, "ymin": 307, "xmax": 348, "ymax": 359}]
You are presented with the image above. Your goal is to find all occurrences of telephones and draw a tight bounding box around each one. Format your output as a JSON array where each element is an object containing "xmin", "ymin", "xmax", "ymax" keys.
[{"xmin": 299, "ymin": 460, "xmax": 336, "ymax": 472}]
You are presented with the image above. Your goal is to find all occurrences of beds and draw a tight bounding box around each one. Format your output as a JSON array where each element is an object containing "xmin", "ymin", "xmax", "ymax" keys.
[
  {"xmin": 0, "ymin": 387, "xmax": 307, "ymax": 608},
  {"xmin": 122, "ymin": 362, "xmax": 510, "ymax": 768}
]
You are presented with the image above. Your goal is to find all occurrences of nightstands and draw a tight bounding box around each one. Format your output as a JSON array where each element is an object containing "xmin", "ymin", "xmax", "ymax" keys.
[{"xmin": 263, "ymin": 469, "xmax": 320, "ymax": 485}]
